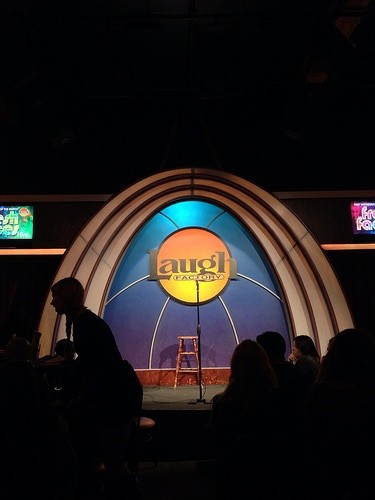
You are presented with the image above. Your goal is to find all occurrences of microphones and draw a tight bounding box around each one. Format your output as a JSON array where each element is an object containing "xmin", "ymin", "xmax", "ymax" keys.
[{"xmin": 195, "ymin": 279, "xmax": 199, "ymax": 289}]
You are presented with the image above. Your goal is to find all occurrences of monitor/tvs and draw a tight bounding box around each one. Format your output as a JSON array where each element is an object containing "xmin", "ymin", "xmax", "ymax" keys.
[
  {"xmin": 346, "ymin": 199, "xmax": 375, "ymax": 243},
  {"xmin": 0, "ymin": 201, "xmax": 43, "ymax": 248}
]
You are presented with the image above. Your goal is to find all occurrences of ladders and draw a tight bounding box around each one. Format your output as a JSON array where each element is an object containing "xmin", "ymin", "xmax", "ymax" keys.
[{"xmin": 173, "ymin": 335, "xmax": 204, "ymax": 391}]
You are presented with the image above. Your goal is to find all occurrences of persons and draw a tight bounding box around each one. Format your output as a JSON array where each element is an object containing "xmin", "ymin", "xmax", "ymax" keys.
[
  {"xmin": 255, "ymin": 332, "xmax": 335, "ymax": 387},
  {"xmin": 52, "ymin": 277, "xmax": 141, "ymax": 495},
  {"xmin": 0, "ymin": 329, "xmax": 74, "ymax": 406},
  {"xmin": 213, "ymin": 340, "xmax": 286, "ymax": 500},
  {"xmin": 305, "ymin": 329, "xmax": 375, "ymax": 500}
]
